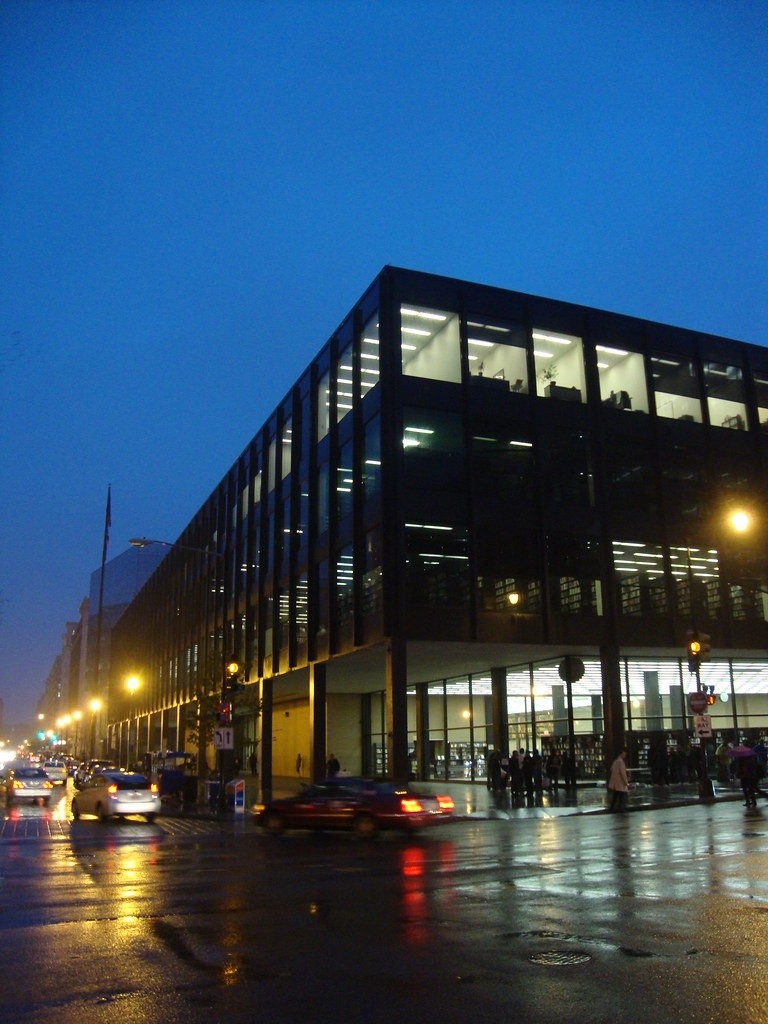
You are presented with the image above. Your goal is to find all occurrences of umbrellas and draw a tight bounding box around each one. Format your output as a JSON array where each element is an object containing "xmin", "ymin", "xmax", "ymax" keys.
[{"xmin": 722, "ymin": 746, "xmax": 757, "ymax": 759}]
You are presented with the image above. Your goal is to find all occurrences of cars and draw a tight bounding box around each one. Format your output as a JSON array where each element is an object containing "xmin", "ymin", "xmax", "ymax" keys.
[
  {"xmin": 2, "ymin": 767, "xmax": 54, "ymax": 804},
  {"xmin": 71, "ymin": 771, "xmax": 161, "ymax": 823},
  {"xmin": 14, "ymin": 746, "xmax": 126, "ymax": 790},
  {"xmin": 41, "ymin": 762, "xmax": 69, "ymax": 786},
  {"xmin": 250, "ymin": 775, "xmax": 456, "ymax": 838}
]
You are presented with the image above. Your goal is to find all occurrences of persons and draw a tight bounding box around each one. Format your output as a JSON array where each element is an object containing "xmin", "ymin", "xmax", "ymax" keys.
[
  {"xmin": 326, "ymin": 753, "xmax": 340, "ymax": 779},
  {"xmin": 486, "ymin": 747, "xmax": 571, "ymax": 797},
  {"xmin": 250, "ymin": 752, "xmax": 257, "ymax": 775},
  {"xmin": 648, "ymin": 739, "xmax": 768, "ymax": 807},
  {"xmin": 606, "ymin": 750, "xmax": 630, "ymax": 812}
]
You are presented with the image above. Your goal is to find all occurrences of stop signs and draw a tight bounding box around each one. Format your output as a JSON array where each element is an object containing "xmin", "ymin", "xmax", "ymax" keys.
[{"xmin": 689, "ymin": 692, "xmax": 708, "ymax": 714}]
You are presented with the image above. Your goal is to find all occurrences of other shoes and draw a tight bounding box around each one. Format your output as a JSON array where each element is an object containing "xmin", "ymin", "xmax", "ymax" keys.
[
  {"xmin": 751, "ymin": 801, "xmax": 756, "ymax": 806},
  {"xmin": 743, "ymin": 801, "xmax": 751, "ymax": 806}
]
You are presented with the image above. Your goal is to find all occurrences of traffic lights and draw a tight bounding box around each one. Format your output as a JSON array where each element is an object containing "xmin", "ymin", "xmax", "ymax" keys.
[
  {"xmin": 699, "ymin": 631, "xmax": 712, "ymax": 664},
  {"xmin": 687, "ymin": 630, "xmax": 700, "ymax": 665},
  {"xmin": 227, "ymin": 657, "xmax": 239, "ymax": 692},
  {"xmin": 707, "ymin": 693, "xmax": 717, "ymax": 703}
]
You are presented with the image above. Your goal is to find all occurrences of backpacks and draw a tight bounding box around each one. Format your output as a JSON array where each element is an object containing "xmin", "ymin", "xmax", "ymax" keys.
[{"xmin": 749, "ymin": 764, "xmax": 763, "ymax": 779}]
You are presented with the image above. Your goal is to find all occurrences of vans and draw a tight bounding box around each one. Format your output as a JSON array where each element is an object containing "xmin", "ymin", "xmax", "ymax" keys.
[{"xmin": 131, "ymin": 750, "xmax": 198, "ymax": 802}]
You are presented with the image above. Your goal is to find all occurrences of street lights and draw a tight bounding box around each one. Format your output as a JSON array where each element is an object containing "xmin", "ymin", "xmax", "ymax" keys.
[{"xmin": 131, "ymin": 533, "xmax": 230, "ymax": 816}]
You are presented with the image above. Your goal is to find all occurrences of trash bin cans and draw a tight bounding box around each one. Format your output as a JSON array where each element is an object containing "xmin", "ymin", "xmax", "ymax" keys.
[{"xmin": 225, "ymin": 778, "xmax": 245, "ymax": 815}]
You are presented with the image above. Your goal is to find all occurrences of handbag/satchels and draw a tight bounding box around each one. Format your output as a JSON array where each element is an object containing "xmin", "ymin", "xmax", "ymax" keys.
[{"xmin": 500, "ymin": 767, "xmax": 507, "ymax": 779}]
